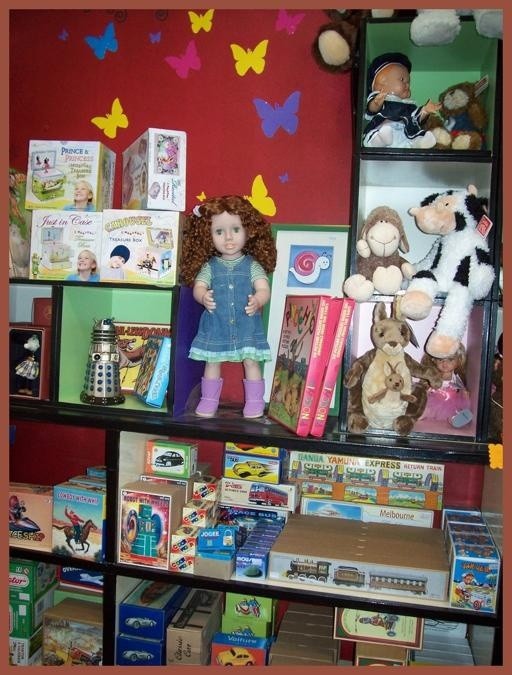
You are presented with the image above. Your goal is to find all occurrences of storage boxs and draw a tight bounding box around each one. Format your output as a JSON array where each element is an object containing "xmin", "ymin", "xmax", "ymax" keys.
[
  {"xmin": 9, "ymin": 465, "xmax": 106, "ymax": 666},
  {"xmin": 116, "ymin": 580, "xmax": 288, "ymax": 666},
  {"xmin": 9, "ymin": 128, "xmax": 187, "ymax": 287}
]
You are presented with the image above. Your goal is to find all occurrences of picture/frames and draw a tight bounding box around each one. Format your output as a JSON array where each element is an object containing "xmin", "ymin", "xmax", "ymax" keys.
[{"xmin": 259, "ymin": 222, "xmax": 351, "ymax": 418}]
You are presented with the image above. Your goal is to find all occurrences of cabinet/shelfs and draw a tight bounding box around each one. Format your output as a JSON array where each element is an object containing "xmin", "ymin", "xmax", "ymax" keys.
[
  {"xmin": 340, "ymin": 7, "xmax": 502, "ymax": 449},
  {"xmin": 8, "ymin": 398, "xmax": 503, "ymax": 667},
  {"xmin": 8, "ymin": 277, "xmax": 206, "ymax": 419}
]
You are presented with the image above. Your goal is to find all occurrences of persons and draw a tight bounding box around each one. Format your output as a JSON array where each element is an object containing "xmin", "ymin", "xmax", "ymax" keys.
[
  {"xmin": 177, "ymin": 192, "xmax": 278, "ymax": 423},
  {"xmin": 65, "ymin": 247, "xmax": 102, "ymax": 284},
  {"xmin": 284, "ymin": 324, "xmax": 313, "ymax": 401},
  {"xmin": 365, "ymin": 48, "xmax": 444, "ymax": 153},
  {"xmin": 404, "ymin": 332, "xmax": 474, "ymax": 433},
  {"xmin": 102, "ymin": 245, "xmax": 132, "ymax": 284},
  {"xmin": 63, "ymin": 179, "xmax": 98, "ymax": 213},
  {"xmin": 136, "ymin": 139, "xmax": 148, "ymax": 163},
  {"xmin": 64, "ymin": 503, "xmax": 86, "ymax": 546}
]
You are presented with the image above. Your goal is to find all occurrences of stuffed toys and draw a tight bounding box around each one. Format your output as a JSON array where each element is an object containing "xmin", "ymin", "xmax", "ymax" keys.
[
  {"xmin": 340, "ymin": 301, "xmax": 433, "ymax": 441},
  {"xmin": 341, "ymin": 203, "xmax": 416, "ymax": 306},
  {"xmin": 432, "ymin": 76, "xmax": 487, "ymax": 154},
  {"xmin": 392, "ymin": 181, "xmax": 499, "ymax": 365}
]
